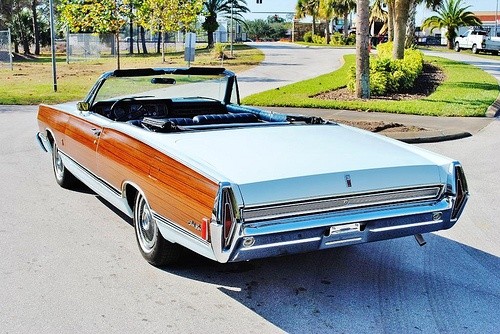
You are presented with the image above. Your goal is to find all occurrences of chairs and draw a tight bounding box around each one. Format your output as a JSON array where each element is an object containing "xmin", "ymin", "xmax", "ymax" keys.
[
  {"xmin": 193, "ymin": 112, "xmax": 258, "ymax": 124},
  {"xmin": 124, "ymin": 119, "xmax": 193, "ymax": 131}
]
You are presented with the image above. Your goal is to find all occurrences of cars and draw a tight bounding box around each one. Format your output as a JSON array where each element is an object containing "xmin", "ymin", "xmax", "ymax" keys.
[{"xmin": 36, "ymin": 67, "xmax": 469, "ymax": 272}]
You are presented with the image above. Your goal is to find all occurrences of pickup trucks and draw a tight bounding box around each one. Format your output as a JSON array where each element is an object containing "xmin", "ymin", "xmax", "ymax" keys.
[{"xmin": 454, "ymin": 29, "xmax": 500, "ymax": 58}]
